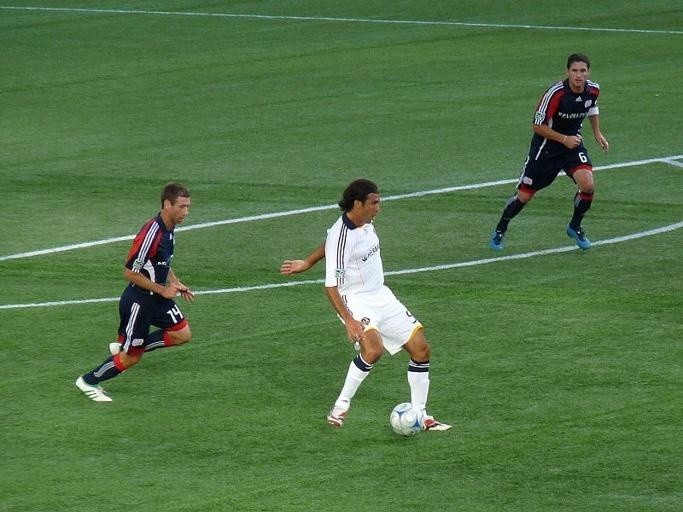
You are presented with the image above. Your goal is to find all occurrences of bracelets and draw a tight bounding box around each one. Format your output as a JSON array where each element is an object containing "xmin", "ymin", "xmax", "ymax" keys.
[{"xmin": 561, "ymin": 136, "xmax": 567, "ymax": 145}]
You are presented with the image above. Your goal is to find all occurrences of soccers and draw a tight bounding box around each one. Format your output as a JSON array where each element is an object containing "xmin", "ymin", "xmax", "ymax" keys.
[{"xmin": 389, "ymin": 402, "xmax": 424, "ymax": 437}]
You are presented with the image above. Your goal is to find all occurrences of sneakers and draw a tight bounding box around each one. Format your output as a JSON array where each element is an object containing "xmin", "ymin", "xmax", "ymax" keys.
[
  {"xmin": 109, "ymin": 343, "xmax": 121, "ymax": 355},
  {"xmin": 422, "ymin": 416, "xmax": 453, "ymax": 431},
  {"xmin": 491, "ymin": 229, "xmax": 504, "ymax": 249},
  {"xmin": 75, "ymin": 376, "xmax": 112, "ymax": 402},
  {"xmin": 327, "ymin": 410, "xmax": 346, "ymax": 427},
  {"xmin": 567, "ymin": 223, "xmax": 590, "ymax": 250}
]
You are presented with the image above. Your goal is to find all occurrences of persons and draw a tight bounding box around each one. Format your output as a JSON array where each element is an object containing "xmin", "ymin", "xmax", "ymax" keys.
[
  {"xmin": 489, "ymin": 53, "xmax": 609, "ymax": 251},
  {"xmin": 279, "ymin": 177, "xmax": 453, "ymax": 433},
  {"xmin": 74, "ymin": 182, "xmax": 196, "ymax": 402}
]
